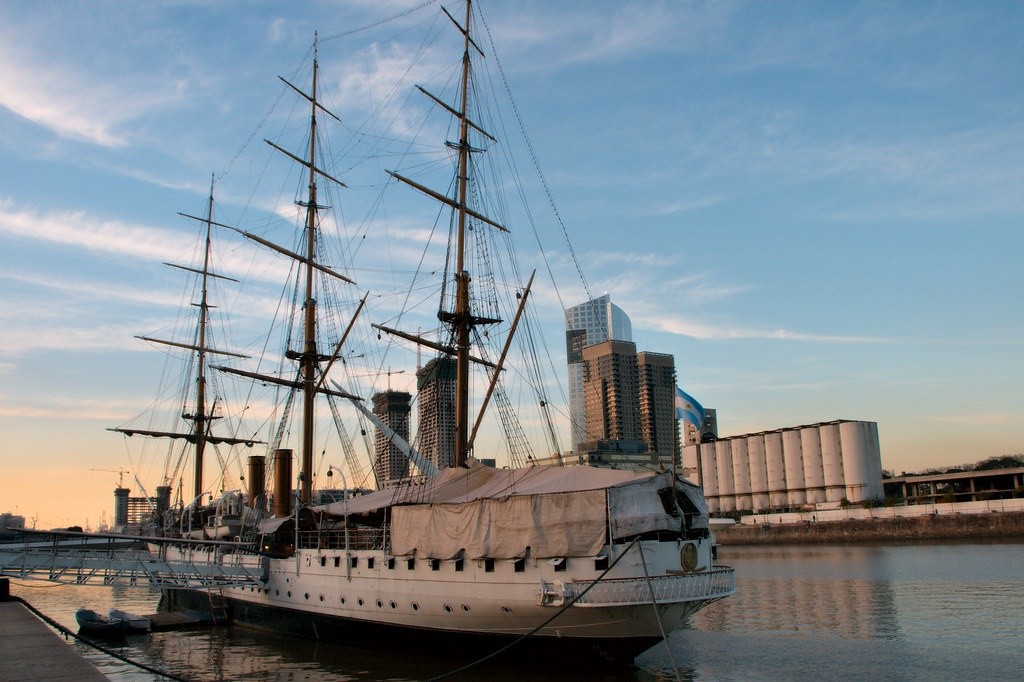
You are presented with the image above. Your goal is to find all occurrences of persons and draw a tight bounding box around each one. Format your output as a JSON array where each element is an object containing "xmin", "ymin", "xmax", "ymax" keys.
[{"xmin": 762, "ymin": 523, "xmax": 770, "ymax": 530}]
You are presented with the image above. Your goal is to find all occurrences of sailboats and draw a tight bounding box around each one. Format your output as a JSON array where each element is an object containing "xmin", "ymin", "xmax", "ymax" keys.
[{"xmin": 76, "ymin": 0, "xmax": 739, "ymax": 643}]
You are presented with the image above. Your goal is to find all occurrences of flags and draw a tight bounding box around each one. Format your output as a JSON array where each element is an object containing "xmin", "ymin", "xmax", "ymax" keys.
[{"xmin": 675, "ymin": 386, "xmax": 706, "ymax": 430}]
